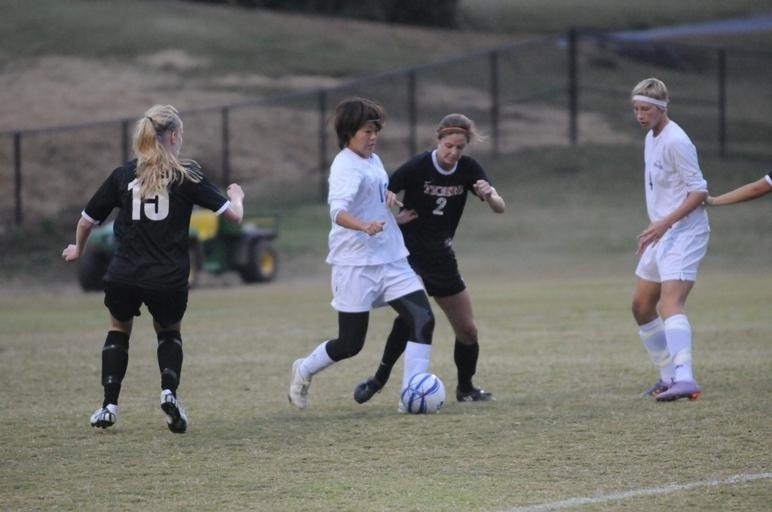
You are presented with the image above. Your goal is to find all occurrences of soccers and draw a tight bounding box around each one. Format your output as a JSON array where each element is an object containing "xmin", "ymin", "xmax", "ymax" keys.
[{"xmin": 399, "ymin": 371, "xmax": 447, "ymax": 415}]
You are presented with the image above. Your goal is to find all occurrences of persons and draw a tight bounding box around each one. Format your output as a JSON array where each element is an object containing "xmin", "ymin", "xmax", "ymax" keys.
[
  {"xmin": 629, "ymin": 77, "xmax": 712, "ymax": 402},
  {"xmin": 287, "ymin": 98, "xmax": 436, "ymax": 415},
  {"xmin": 703, "ymin": 172, "xmax": 772, "ymax": 205},
  {"xmin": 61, "ymin": 103, "xmax": 246, "ymax": 434},
  {"xmin": 353, "ymin": 113, "xmax": 506, "ymax": 405}
]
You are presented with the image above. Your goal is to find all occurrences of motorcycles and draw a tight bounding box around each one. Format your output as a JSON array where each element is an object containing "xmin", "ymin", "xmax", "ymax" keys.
[{"xmin": 63, "ymin": 206, "xmax": 278, "ymax": 286}]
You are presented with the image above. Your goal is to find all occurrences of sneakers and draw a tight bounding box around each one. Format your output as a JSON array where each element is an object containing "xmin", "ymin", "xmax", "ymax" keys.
[
  {"xmin": 354, "ymin": 376, "xmax": 386, "ymax": 404},
  {"xmin": 287, "ymin": 359, "xmax": 313, "ymax": 410},
  {"xmin": 89, "ymin": 403, "xmax": 119, "ymax": 429},
  {"xmin": 160, "ymin": 388, "xmax": 189, "ymax": 434},
  {"xmin": 457, "ymin": 382, "xmax": 494, "ymax": 403},
  {"xmin": 638, "ymin": 378, "xmax": 702, "ymax": 402}
]
